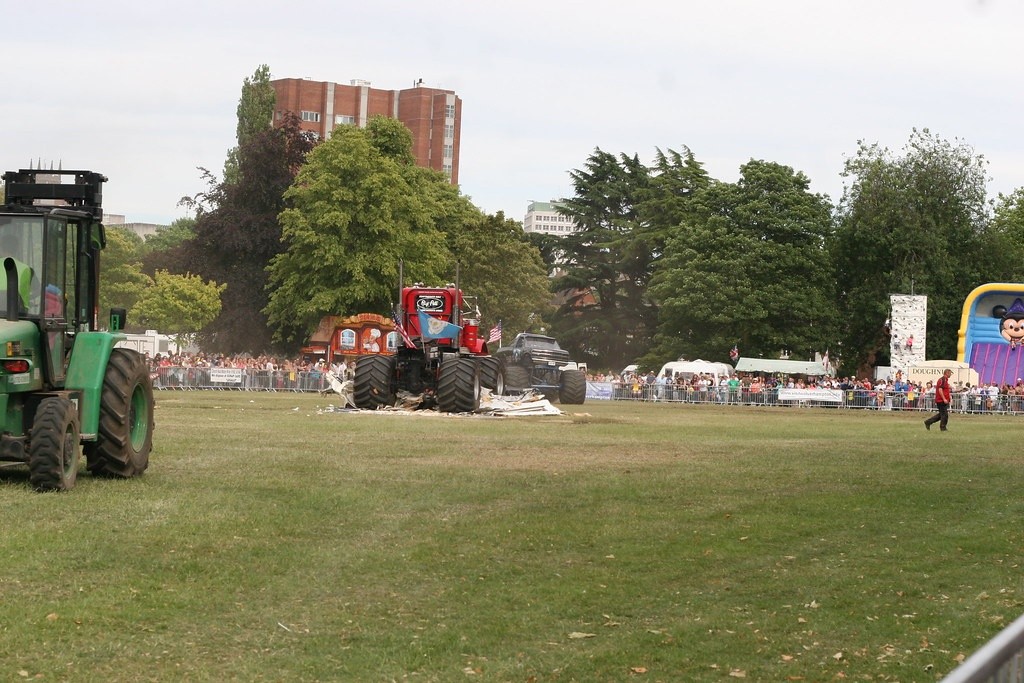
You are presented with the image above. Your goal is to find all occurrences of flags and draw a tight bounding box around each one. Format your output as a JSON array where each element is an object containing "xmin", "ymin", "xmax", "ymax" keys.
[
  {"xmin": 487, "ymin": 320, "xmax": 502, "ymax": 345},
  {"xmin": 418, "ymin": 305, "xmax": 463, "ymax": 355},
  {"xmin": 391, "ymin": 302, "xmax": 417, "ymax": 348}
]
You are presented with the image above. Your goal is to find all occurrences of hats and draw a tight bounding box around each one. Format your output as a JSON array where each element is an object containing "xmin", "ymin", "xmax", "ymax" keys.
[
  {"xmin": 753, "ymin": 378, "xmax": 757, "ymax": 381},
  {"xmin": 725, "ymin": 374, "xmax": 729, "ymax": 378},
  {"xmin": 851, "ymin": 376, "xmax": 855, "ymax": 378},
  {"xmin": 1017, "ymin": 378, "xmax": 1021, "ymax": 380},
  {"xmin": 649, "ymin": 371, "xmax": 655, "ymax": 375},
  {"xmin": 719, "ymin": 375, "xmax": 724, "ymax": 376}
]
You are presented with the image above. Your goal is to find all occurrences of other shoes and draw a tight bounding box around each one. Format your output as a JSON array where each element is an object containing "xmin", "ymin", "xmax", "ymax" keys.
[
  {"xmin": 924, "ymin": 421, "xmax": 930, "ymax": 430},
  {"xmin": 940, "ymin": 428, "xmax": 947, "ymax": 431}
]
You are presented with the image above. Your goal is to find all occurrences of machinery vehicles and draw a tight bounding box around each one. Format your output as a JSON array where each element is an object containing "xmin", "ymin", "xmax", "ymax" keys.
[
  {"xmin": 494, "ymin": 331, "xmax": 589, "ymax": 405},
  {"xmin": 351, "ymin": 256, "xmax": 503, "ymax": 412},
  {"xmin": 0, "ymin": 168, "xmax": 156, "ymax": 491}
]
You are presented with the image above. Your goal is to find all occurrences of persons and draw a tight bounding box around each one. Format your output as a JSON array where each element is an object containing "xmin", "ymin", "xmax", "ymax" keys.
[
  {"xmin": 146, "ymin": 349, "xmax": 356, "ymax": 388},
  {"xmin": 924, "ymin": 369, "xmax": 952, "ymax": 430},
  {"xmin": 586, "ymin": 369, "xmax": 1023, "ymax": 415},
  {"xmin": 894, "ymin": 333, "xmax": 914, "ymax": 352},
  {"xmin": 362, "ymin": 329, "xmax": 381, "ymax": 352}
]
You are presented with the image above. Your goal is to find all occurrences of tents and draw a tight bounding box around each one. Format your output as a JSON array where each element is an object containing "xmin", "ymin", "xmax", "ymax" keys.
[
  {"xmin": 656, "ymin": 360, "xmax": 733, "ymax": 377},
  {"xmin": 735, "ymin": 357, "xmax": 825, "ymax": 376}
]
